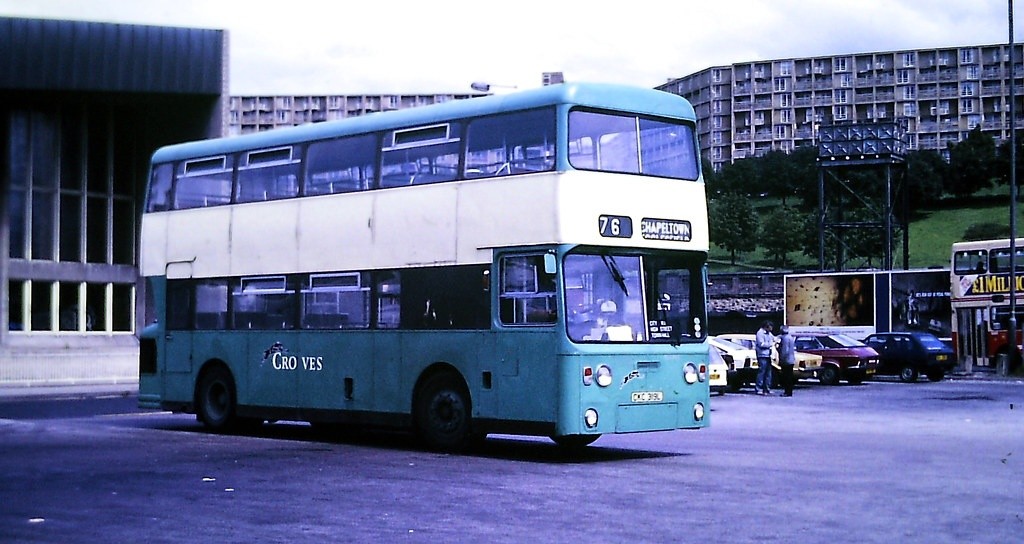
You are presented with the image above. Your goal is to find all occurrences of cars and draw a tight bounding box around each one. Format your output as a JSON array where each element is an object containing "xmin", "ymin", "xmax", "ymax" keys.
[
  {"xmin": 708, "ymin": 333, "xmax": 822, "ymax": 397},
  {"xmin": 789, "ymin": 331, "xmax": 881, "ymax": 385},
  {"xmin": 859, "ymin": 332, "xmax": 953, "ymax": 382}
]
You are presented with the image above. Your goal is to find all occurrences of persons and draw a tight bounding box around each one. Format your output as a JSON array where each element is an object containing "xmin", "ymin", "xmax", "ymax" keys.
[
  {"xmin": 756, "ymin": 321, "xmax": 775, "ymax": 394},
  {"xmin": 906, "ymin": 290, "xmax": 918, "ymax": 324},
  {"xmin": 774, "ymin": 326, "xmax": 796, "ymax": 397}
]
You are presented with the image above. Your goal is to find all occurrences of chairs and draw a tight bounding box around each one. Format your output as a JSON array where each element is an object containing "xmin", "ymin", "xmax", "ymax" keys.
[
  {"xmin": 195, "ymin": 310, "xmax": 351, "ymax": 330},
  {"xmin": 174, "ymin": 162, "xmax": 549, "ymax": 211}
]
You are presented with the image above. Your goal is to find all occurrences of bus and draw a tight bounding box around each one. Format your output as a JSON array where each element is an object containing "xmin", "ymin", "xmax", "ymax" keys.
[
  {"xmin": 950, "ymin": 239, "xmax": 1024, "ymax": 368},
  {"xmin": 132, "ymin": 81, "xmax": 712, "ymax": 448}
]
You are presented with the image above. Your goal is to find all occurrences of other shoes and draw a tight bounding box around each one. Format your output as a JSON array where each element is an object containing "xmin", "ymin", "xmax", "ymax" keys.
[{"xmin": 780, "ymin": 393, "xmax": 792, "ymax": 397}]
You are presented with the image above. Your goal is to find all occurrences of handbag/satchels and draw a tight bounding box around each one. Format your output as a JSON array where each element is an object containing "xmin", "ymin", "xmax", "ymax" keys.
[{"xmin": 771, "ymin": 344, "xmax": 777, "ymax": 359}]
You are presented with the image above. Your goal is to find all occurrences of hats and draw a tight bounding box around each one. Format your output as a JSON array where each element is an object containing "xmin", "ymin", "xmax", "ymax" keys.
[
  {"xmin": 780, "ymin": 325, "xmax": 789, "ymax": 333},
  {"xmin": 601, "ymin": 301, "xmax": 616, "ymax": 312}
]
